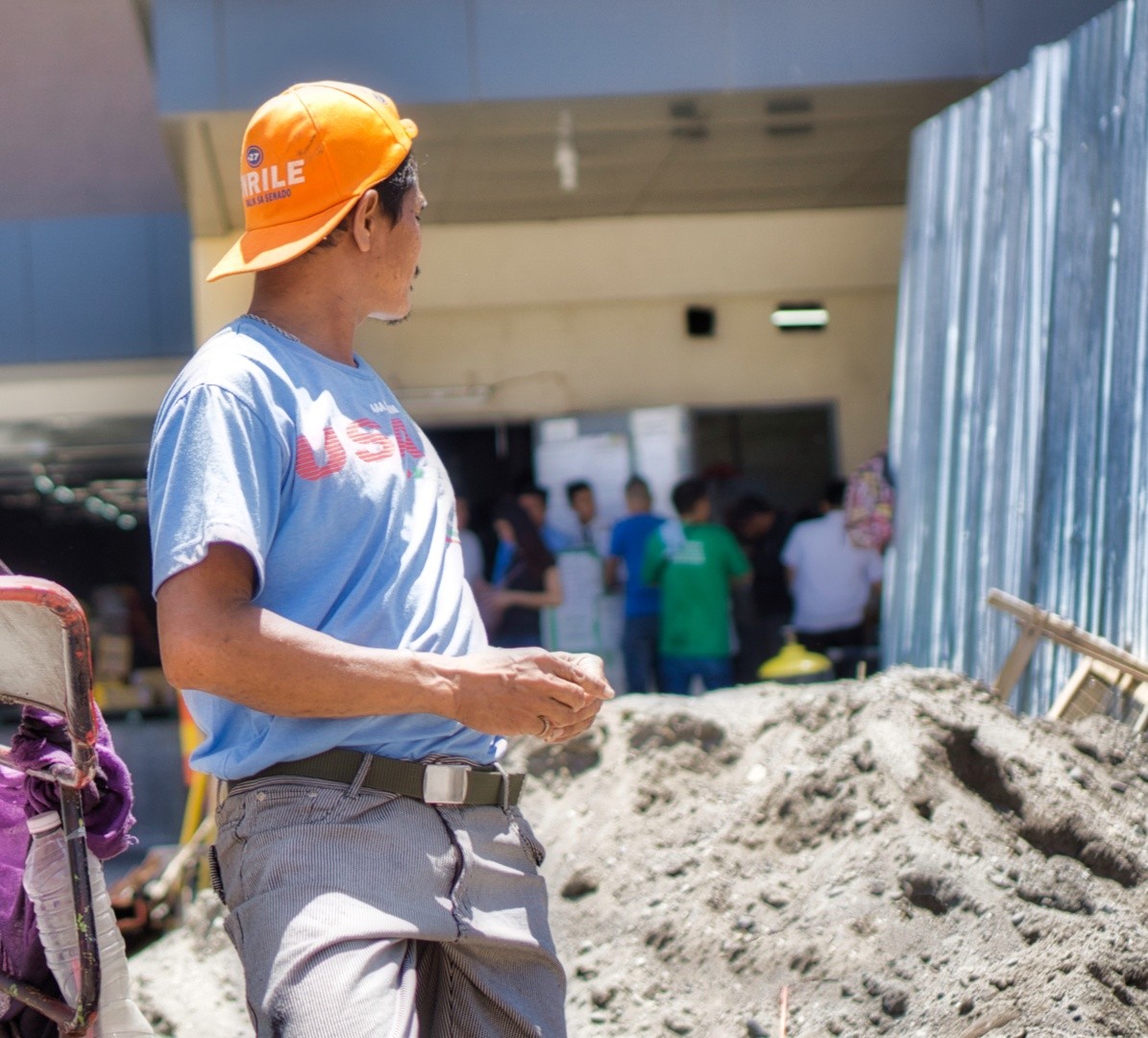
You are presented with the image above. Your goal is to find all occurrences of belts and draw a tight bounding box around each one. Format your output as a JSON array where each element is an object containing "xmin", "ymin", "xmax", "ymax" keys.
[{"xmin": 228, "ymin": 746, "xmax": 529, "ymax": 810}]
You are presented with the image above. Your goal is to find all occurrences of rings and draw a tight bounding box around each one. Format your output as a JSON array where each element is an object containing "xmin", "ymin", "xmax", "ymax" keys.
[{"xmin": 536, "ymin": 717, "xmax": 550, "ymax": 740}]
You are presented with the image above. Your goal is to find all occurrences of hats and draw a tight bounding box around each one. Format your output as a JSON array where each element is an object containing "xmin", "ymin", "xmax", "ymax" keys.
[{"xmin": 206, "ymin": 81, "xmax": 419, "ymax": 283}]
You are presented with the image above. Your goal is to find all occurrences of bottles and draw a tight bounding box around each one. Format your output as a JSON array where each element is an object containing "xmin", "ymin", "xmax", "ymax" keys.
[{"xmin": 22, "ymin": 811, "xmax": 136, "ymax": 1010}]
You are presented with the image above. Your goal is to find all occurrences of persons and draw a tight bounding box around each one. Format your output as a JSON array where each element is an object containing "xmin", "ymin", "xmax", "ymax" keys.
[
  {"xmin": 148, "ymin": 81, "xmax": 616, "ymax": 1037},
  {"xmin": 89, "ymin": 454, "xmax": 893, "ymax": 698}
]
